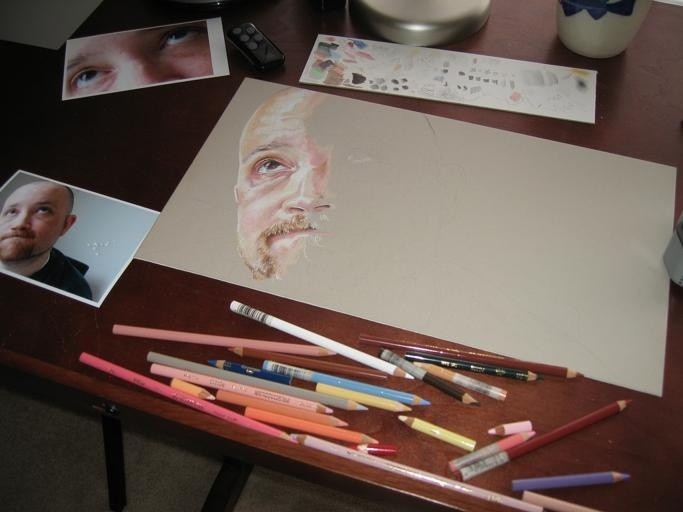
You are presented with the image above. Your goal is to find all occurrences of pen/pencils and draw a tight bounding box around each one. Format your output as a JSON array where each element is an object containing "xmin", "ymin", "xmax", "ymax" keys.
[{"xmin": 79, "ymin": 300, "xmax": 633, "ymax": 512}]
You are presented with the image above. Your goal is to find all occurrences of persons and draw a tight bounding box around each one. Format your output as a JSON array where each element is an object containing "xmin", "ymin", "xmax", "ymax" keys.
[
  {"xmin": 62, "ymin": 20, "xmax": 214, "ymax": 100},
  {"xmin": 233, "ymin": 87, "xmax": 333, "ymax": 280},
  {"xmin": 0, "ymin": 180, "xmax": 93, "ymax": 302}
]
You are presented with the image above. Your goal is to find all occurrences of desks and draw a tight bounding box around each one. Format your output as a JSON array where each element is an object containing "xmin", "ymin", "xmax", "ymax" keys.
[{"xmin": 0, "ymin": 0, "xmax": 682, "ymax": 512}]
